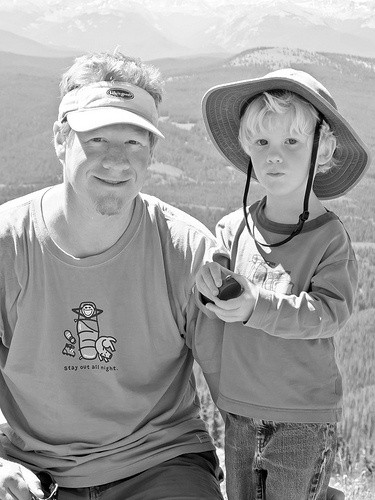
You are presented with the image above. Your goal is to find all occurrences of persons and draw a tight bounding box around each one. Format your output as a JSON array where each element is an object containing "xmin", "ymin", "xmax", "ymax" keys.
[
  {"xmin": 0, "ymin": 49, "xmax": 345, "ymax": 500},
  {"xmin": 186, "ymin": 68, "xmax": 371, "ymax": 500}
]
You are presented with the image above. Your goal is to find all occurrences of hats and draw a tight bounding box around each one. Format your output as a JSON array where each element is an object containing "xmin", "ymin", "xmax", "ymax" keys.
[
  {"xmin": 58, "ymin": 81, "xmax": 165, "ymax": 140},
  {"xmin": 201, "ymin": 68, "xmax": 371, "ymax": 201}
]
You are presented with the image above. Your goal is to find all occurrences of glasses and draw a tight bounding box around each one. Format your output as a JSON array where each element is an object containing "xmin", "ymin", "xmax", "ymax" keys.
[{"xmin": 29, "ymin": 471, "xmax": 59, "ymax": 500}]
